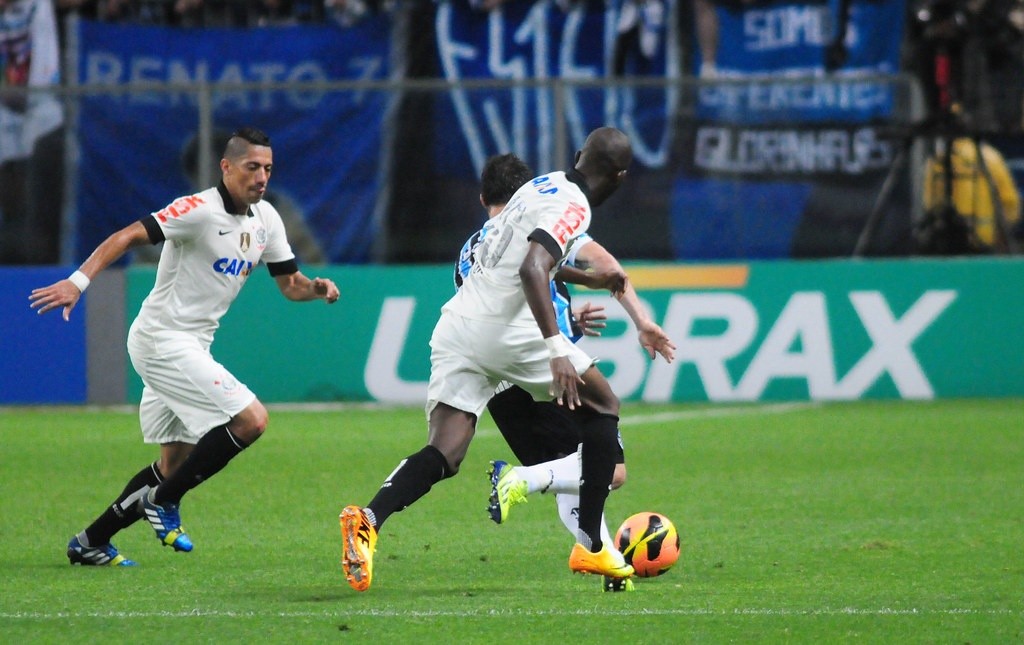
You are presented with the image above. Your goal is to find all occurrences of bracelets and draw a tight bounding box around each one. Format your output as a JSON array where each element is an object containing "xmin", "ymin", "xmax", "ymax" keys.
[{"xmin": 68, "ymin": 270, "xmax": 91, "ymax": 293}]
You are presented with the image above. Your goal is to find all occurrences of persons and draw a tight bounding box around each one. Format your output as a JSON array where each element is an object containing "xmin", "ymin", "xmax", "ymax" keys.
[
  {"xmin": 453, "ymin": 152, "xmax": 677, "ymax": 593},
  {"xmin": 923, "ymin": 104, "xmax": 1021, "ymax": 252},
  {"xmin": 338, "ymin": 127, "xmax": 635, "ymax": 593},
  {"xmin": 28, "ymin": 127, "xmax": 339, "ymax": 567}
]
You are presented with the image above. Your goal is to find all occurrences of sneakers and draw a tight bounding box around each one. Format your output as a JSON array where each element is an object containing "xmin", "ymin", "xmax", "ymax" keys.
[
  {"xmin": 486, "ymin": 459, "xmax": 527, "ymax": 524},
  {"xmin": 601, "ymin": 575, "xmax": 636, "ymax": 591},
  {"xmin": 339, "ymin": 505, "xmax": 378, "ymax": 591},
  {"xmin": 568, "ymin": 539, "xmax": 635, "ymax": 579},
  {"xmin": 67, "ymin": 535, "xmax": 136, "ymax": 566},
  {"xmin": 140, "ymin": 487, "xmax": 193, "ymax": 552}
]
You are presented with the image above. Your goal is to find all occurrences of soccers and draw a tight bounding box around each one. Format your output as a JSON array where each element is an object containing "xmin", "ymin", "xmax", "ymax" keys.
[{"xmin": 614, "ymin": 511, "xmax": 680, "ymax": 578}]
